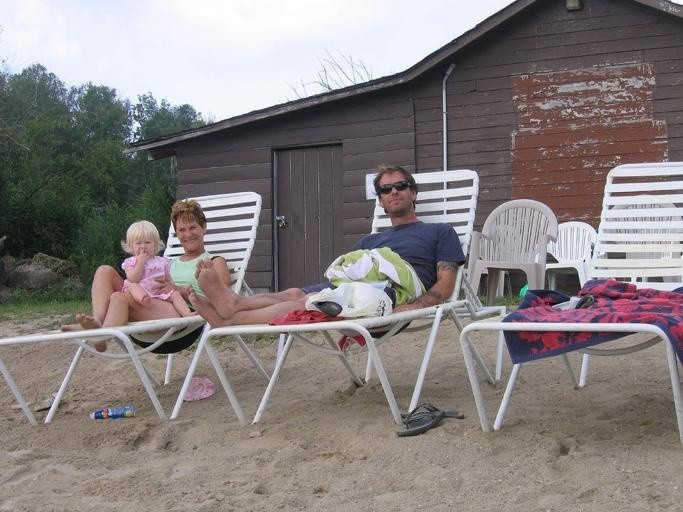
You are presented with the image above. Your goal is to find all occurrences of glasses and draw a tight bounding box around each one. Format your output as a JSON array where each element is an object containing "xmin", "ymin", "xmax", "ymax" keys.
[{"xmin": 377, "ymin": 181, "xmax": 413, "ymax": 195}]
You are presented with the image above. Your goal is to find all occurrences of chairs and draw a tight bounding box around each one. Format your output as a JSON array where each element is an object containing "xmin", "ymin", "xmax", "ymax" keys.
[
  {"xmin": 207, "ymin": 170, "xmax": 480, "ymax": 431},
  {"xmin": 0, "ymin": 192, "xmax": 272, "ymax": 424},
  {"xmin": 462, "ymin": 160, "xmax": 681, "ymax": 442}
]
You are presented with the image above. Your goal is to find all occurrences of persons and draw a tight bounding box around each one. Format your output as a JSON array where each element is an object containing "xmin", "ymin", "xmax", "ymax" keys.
[
  {"xmin": 187, "ymin": 164, "xmax": 465, "ymax": 332},
  {"xmin": 57, "ymin": 194, "xmax": 233, "ymax": 353},
  {"xmin": 118, "ymin": 220, "xmax": 199, "ymax": 318}
]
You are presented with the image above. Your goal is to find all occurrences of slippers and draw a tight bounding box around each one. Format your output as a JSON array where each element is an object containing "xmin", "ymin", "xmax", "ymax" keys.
[{"xmin": 394, "ymin": 401, "xmax": 464, "ymax": 438}]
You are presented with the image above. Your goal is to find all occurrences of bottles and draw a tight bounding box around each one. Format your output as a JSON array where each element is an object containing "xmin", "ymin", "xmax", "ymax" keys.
[{"xmin": 89, "ymin": 405, "xmax": 136, "ymax": 420}]
